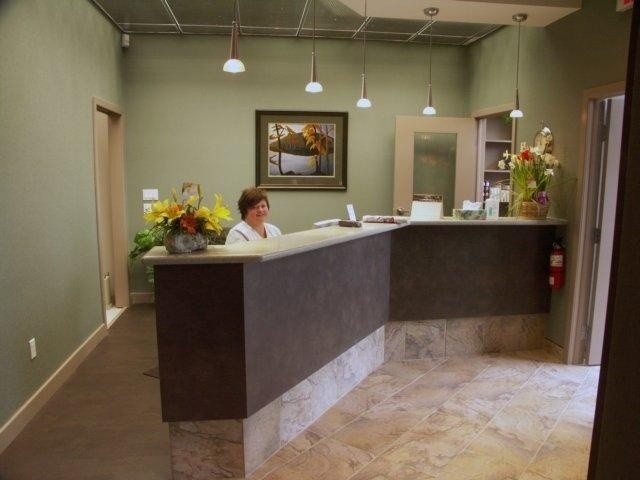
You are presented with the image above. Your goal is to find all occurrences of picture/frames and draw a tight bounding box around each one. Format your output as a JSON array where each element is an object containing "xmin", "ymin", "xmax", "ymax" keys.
[{"xmin": 255, "ymin": 109, "xmax": 348, "ymax": 191}]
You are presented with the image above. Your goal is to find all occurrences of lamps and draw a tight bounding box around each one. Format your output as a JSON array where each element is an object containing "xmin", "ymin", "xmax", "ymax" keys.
[
  {"xmin": 422, "ymin": 8, "xmax": 439, "ymax": 114},
  {"xmin": 305, "ymin": 0, "xmax": 322, "ymax": 92},
  {"xmin": 357, "ymin": 0, "xmax": 371, "ymax": 108},
  {"xmin": 510, "ymin": 14, "xmax": 527, "ymax": 117},
  {"xmin": 223, "ymin": 0, "xmax": 245, "ymax": 73}
]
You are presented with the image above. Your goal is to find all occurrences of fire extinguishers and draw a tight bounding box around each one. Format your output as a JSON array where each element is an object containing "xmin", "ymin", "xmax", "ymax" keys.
[{"xmin": 548, "ymin": 236, "xmax": 567, "ymax": 289}]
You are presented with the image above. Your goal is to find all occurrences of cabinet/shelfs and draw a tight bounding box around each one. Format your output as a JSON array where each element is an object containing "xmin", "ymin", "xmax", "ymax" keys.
[{"xmin": 484, "ymin": 140, "xmax": 511, "ymax": 204}]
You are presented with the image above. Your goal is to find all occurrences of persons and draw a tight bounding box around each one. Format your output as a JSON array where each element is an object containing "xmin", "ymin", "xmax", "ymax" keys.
[{"xmin": 225, "ymin": 187, "xmax": 282, "ymax": 245}]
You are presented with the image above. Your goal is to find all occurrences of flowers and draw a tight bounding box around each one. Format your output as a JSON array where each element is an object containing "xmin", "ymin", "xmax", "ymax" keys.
[
  {"xmin": 128, "ymin": 181, "xmax": 232, "ymax": 260},
  {"xmin": 498, "ymin": 143, "xmax": 577, "ymax": 220}
]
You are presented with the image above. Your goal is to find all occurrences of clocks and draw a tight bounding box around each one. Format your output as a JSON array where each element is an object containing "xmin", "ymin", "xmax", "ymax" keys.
[{"xmin": 533, "ymin": 119, "xmax": 553, "ymax": 154}]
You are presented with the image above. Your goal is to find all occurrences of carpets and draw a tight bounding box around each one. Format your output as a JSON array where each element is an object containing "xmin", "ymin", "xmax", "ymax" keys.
[{"xmin": 143, "ymin": 367, "xmax": 160, "ymax": 378}]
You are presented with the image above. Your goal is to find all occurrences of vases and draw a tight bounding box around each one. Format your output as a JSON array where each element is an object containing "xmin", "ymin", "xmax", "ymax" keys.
[
  {"xmin": 164, "ymin": 228, "xmax": 207, "ymax": 253},
  {"xmin": 519, "ymin": 202, "xmax": 551, "ymax": 219}
]
